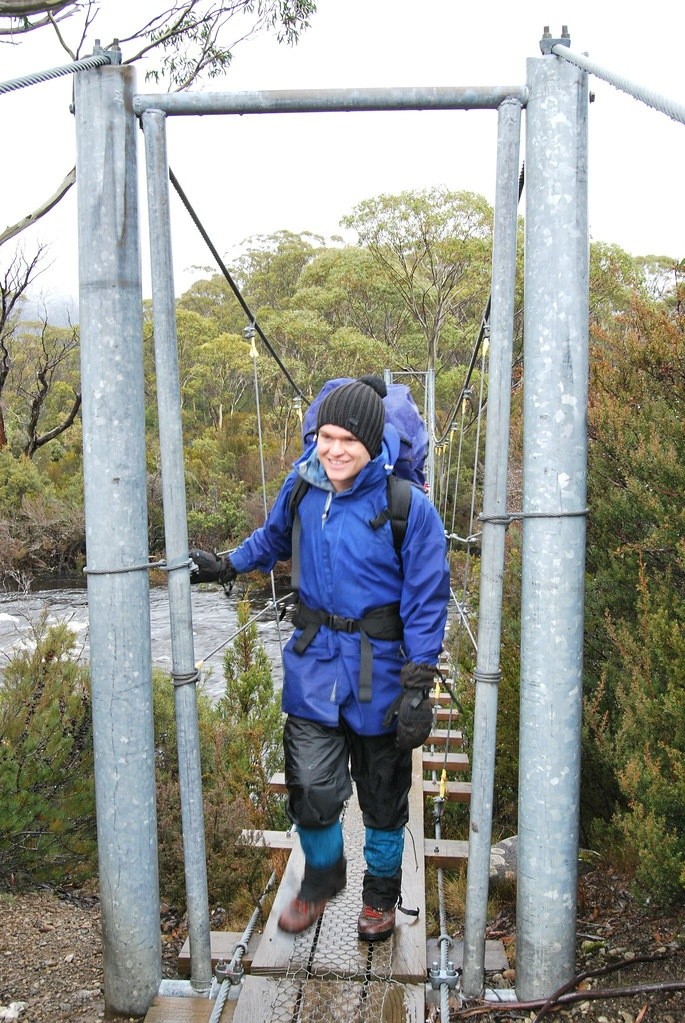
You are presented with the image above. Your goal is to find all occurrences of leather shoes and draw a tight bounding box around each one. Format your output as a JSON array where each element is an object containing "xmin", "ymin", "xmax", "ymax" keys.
[
  {"xmin": 357, "ymin": 902, "xmax": 396, "ymax": 941},
  {"xmin": 279, "ymin": 898, "xmax": 323, "ymax": 931}
]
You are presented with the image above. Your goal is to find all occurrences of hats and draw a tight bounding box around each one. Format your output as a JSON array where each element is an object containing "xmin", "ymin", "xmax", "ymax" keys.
[{"xmin": 317, "ymin": 376, "xmax": 388, "ymax": 460}]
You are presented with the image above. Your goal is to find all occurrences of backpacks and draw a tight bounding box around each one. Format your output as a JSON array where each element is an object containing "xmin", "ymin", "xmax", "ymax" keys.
[{"xmin": 289, "ymin": 378, "xmax": 431, "ymax": 580}]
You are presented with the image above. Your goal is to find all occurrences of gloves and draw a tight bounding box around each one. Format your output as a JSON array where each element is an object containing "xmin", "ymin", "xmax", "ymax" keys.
[
  {"xmin": 394, "ymin": 665, "xmax": 436, "ymax": 751},
  {"xmin": 187, "ymin": 548, "xmax": 237, "ymax": 585}
]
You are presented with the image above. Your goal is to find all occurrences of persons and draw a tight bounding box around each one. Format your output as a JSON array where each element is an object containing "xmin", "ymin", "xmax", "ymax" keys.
[{"xmin": 188, "ymin": 375, "xmax": 451, "ymax": 941}]
